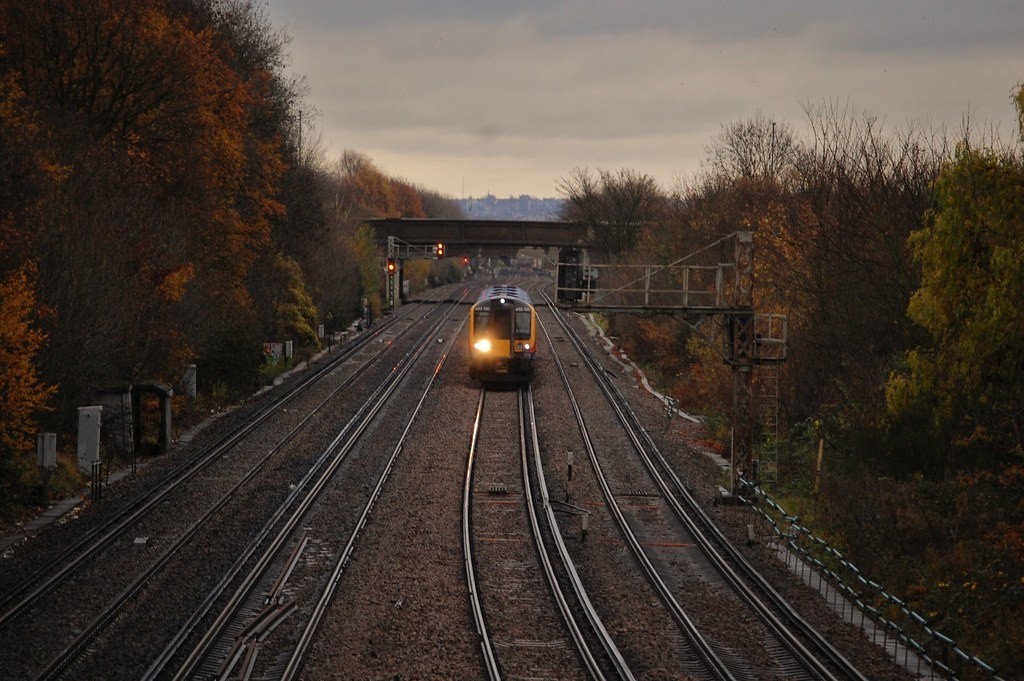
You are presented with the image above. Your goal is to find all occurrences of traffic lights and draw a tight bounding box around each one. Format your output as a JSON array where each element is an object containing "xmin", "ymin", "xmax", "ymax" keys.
[
  {"xmin": 388, "ymin": 258, "xmax": 395, "ymax": 272},
  {"xmin": 464, "ymin": 257, "xmax": 469, "ymax": 263},
  {"xmin": 438, "ymin": 242, "xmax": 444, "ymax": 257}
]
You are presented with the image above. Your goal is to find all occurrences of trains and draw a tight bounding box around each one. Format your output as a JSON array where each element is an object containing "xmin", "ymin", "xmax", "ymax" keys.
[{"xmin": 468, "ymin": 284, "xmax": 536, "ymax": 390}]
